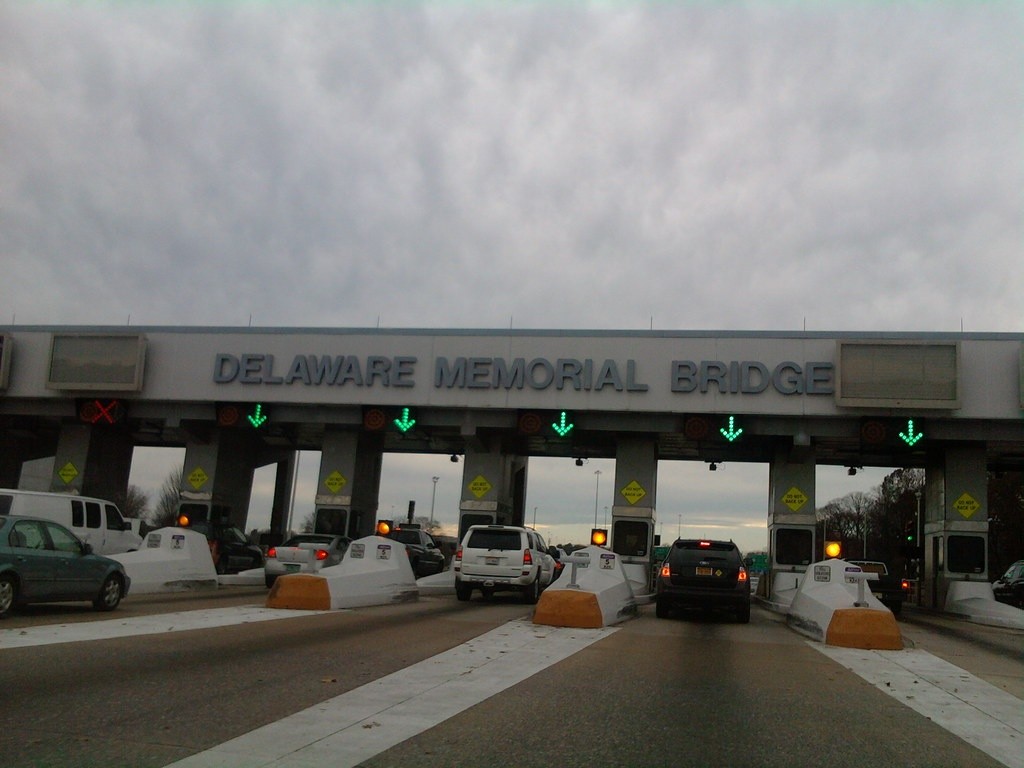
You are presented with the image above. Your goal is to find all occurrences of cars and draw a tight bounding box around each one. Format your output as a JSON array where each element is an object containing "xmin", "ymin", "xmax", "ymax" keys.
[
  {"xmin": 0, "ymin": 515, "xmax": 132, "ymax": 614},
  {"xmin": 547, "ymin": 548, "xmax": 568, "ymax": 585},
  {"xmin": 992, "ymin": 560, "xmax": 1024, "ymax": 609},
  {"xmin": 840, "ymin": 558, "xmax": 909, "ymax": 615},
  {"xmin": 264, "ymin": 534, "xmax": 357, "ymax": 588},
  {"xmin": 192, "ymin": 519, "xmax": 263, "ymax": 575}
]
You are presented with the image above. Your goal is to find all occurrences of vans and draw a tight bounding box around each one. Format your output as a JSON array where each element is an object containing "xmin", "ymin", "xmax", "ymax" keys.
[{"xmin": 1, "ymin": 489, "xmax": 143, "ymax": 557}]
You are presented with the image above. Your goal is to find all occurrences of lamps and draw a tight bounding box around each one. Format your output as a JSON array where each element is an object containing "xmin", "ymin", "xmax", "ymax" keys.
[
  {"xmin": 166, "ymin": 416, "xmax": 181, "ymax": 426},
  {"xmin": 794, "ymin": 431, "xmax": 811, "ymax": 446},
  {"xmin": 572, "ymin": 454, "xmax": 589, "ymax": 466},
  {"xmin": 460, "ymin": 425, "xmax": 476, "ymax": 436},
  {"xmin": 448, "ymin": 453, "xmax": 461, "ymax": 463},
  {"xmin": 704, "ymin": 461, "xmax": 722, "ymax": 471},
  {"xmin": 844, "ymin": 465, "xmax": 863, "ymax": 476}
]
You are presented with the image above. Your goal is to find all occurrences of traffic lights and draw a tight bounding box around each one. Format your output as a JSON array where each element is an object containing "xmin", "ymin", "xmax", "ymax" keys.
[
  {"xmin": 177, "ymin": 513, "xmax": 192, "ymax": 530},
  {"xmin": 591, "ymin": 528, "xmax": 609, "ymax": 547},
  {"xmin": 375, "ymin": 519, "xmax": 393, "ymax": 538},
  {"xmin": 824, "ymin": 541, "xmax": 842, "ymax": 560},
  {"xmin": 904, "ymin": 519, "xmax": 915, "ymax": 547}
]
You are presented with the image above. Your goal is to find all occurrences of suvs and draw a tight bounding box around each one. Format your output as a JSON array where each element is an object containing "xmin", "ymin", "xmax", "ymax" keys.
[
  {"xmin": 454, "ymin": 523, "xmax": 559, "ymax": 606},
  {"xmin": 391, "ymin": 528, "xmax": 446, "ymax": 578},
  {"xmin": 654, "ymin": 537, "xmax": 751, "ymax": 624}
]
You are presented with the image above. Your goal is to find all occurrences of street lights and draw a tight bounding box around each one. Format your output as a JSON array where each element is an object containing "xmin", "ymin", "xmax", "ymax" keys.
[
  {"xmin": 594, "ymin": 469, "xmax": 602, "ymax": 527},
  {"xmin": 660, "ymin": 521, "xmax": 663, "ymax": 545},
  {"xmin": 603, "ymin": 506, "xmax": 608, "ymax": 528},
  {"xmin": 428, "ymin": 476, "xmax": 439, "ymax": 531},
  {"xmin": 678, "ymin": 514, "xmax": 683, "ymax": 538},
  {"xmin": 533, "ymin": 507, "xmax": 538, "ymax": 530}
]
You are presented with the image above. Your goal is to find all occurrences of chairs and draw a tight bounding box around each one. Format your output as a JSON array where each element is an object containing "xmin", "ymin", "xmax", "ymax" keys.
[{"xmin": 9, "ymin": 531, "xmax": 27, "ymax": 547}]
